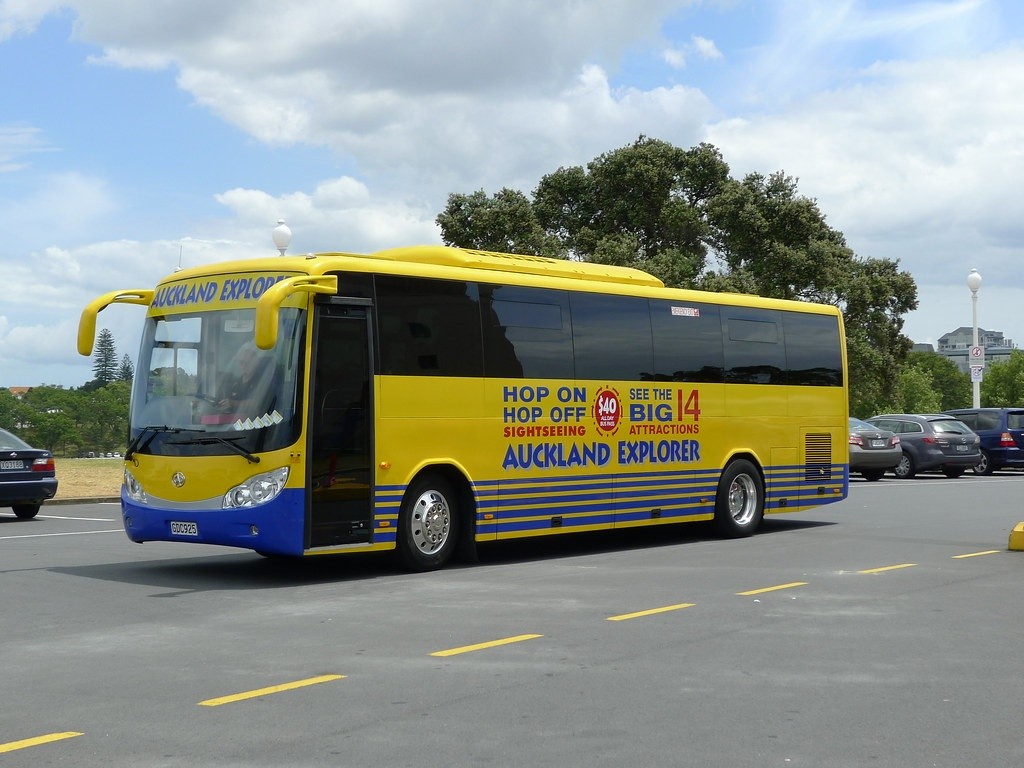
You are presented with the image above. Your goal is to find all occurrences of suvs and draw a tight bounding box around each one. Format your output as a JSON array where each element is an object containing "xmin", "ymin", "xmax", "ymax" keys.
[
  {"xmin": 857, "ymin": 413, "xmax": 983, "ymax": 479},
  {"xmin": 936, "ymin": 407, "xmax": 1024, "ymax": 476}
]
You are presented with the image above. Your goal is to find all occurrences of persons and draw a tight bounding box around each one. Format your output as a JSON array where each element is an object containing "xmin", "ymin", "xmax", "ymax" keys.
[{"xmin": 219, "ymin": 346, "xmax": 277, "ymax": 415}]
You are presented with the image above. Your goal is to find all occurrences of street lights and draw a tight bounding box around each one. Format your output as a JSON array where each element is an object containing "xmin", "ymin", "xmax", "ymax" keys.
[{"xmin": 967, "ymin": 267, "xmax": 983, "ymax": 408}]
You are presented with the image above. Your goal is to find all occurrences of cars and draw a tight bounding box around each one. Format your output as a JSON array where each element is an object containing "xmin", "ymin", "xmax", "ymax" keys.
[
  {"xmin": 848, "ymin": 416, "xmax": 903, "ymax": 482},
  {"xmin": 0, "ymin": 427, "xmax": 59, "ymax": 520}
]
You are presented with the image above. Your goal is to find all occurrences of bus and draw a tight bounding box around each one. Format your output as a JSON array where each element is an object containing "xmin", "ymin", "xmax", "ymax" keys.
[{"xmin": 75, "ymin": 245, "xmax": 849, "ymax": 572}]
